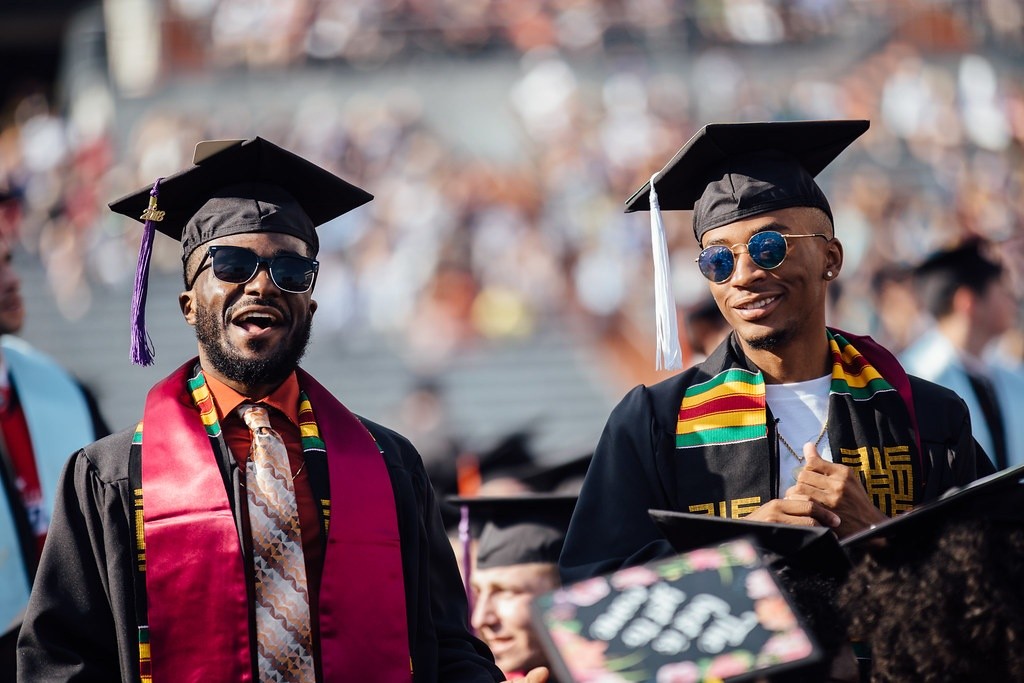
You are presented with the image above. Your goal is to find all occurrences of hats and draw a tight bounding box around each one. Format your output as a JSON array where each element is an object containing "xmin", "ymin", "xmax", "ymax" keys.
[
  {"xmin": 624, "ymin": 119, "xmax": 871, "ymax": 371},
  {"xmin": 445, "ymin": 497, "xmax": 578, "ymax": 635},
  {"xmin": 912, "ymin": 236, "xmax": 990, "ymax": 277},
  {"xmin": 108, "ymin": 135, "xmax": 374, "ymax": 367}
]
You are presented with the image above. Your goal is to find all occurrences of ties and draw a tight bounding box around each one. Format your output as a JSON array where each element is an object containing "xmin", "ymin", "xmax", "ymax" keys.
[{"xmin": 235, "ymin": 404, "xmax": 316, "ymax": 683}]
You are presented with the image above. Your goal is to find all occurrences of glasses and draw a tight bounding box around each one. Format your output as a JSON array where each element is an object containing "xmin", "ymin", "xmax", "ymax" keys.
[
  {"xmin": 694, "ymin": 230, "xmax": 828, "ymax": 284},
  {"xmin": 189, "ymin": 244, "xmax": 320, "ymax": 294}
]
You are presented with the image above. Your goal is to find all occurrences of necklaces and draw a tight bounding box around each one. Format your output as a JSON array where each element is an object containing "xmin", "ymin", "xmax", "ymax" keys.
[{"xmin": 778, "ymin": 420, "xmax": 828, "ymax": 462}]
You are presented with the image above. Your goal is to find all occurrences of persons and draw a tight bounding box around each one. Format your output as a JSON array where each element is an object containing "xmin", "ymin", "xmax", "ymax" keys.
[
  {"xmin": 554, "ymin": 122, "xmax": 998, "ymax": 587},
  {"xmin": 390, "ymin": 229, "xmax": 1023, "ymax": 683},
  {"xmin": 0, "ymin": 184, "xmax": 114, "ymax": 683},
  {"xmin": 16, "ymin": 136, "xmax": 547, "ymax": 682}
]
